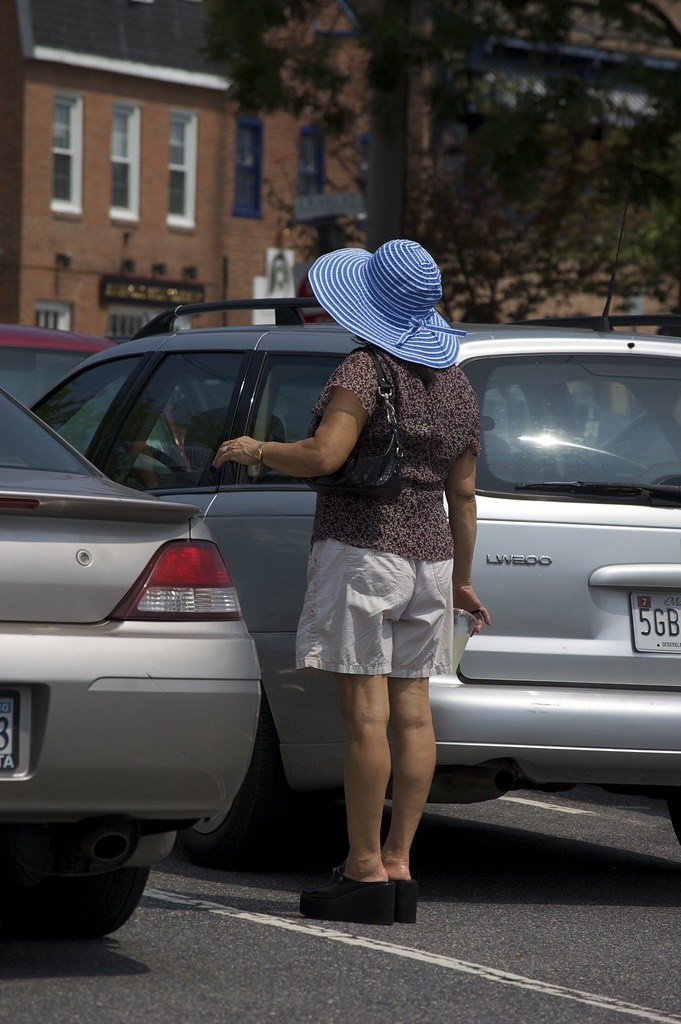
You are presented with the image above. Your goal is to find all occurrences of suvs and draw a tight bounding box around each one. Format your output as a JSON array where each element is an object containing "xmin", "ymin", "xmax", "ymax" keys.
[{"xmin": 27, "ymin": 298, "xmax": 681, "ymax": 870}]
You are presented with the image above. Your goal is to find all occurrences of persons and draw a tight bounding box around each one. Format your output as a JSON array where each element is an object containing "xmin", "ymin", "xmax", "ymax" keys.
[{"xmin": 211, "ymin": 239, "xmax": 492, "ymax": 926}]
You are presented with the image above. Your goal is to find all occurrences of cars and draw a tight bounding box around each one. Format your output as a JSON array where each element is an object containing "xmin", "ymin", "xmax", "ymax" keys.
[
  {"xmin": 0, "ymin": 387, "xmax": 266, "ymax": 935},
  {"xmin": 0, "ymin": 324, "xmax": 120, "ymax": 407}
]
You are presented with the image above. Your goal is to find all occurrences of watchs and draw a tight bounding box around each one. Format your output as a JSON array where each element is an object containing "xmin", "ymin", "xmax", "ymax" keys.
[{"xmin": 254, "ymin": 442, "xmax": 267, "ymax": 464}]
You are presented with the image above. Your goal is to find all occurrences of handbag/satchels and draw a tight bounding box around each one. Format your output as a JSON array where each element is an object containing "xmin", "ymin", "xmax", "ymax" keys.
[{"xmin": 303, "ymin": 347, "xmax": 404, "ymax": 499}]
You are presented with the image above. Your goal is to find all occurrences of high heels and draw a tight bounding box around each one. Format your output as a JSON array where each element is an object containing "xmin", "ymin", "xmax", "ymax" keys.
[{"xmin": 300, "ymin": 859, "xmax": 418, "ymax": 926}]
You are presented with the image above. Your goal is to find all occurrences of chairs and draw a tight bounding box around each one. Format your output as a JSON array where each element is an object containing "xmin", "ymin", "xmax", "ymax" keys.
[{"xmin": 186, "ymin": 406, "xmax": 288, "ymax": 477}]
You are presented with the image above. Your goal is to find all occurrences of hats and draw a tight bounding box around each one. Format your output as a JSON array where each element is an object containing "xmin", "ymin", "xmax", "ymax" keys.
[{"xmin": 308, "ymin": 238, "xmax": 468, "ymax": 369}]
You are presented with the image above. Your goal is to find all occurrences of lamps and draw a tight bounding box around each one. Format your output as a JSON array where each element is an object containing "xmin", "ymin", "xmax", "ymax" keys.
[
  {"xmin": 151, "ymin": 263, "xmax": 171, "ymax": 278},
  {"xmin": 120, "ymin": 258, "xmax": 136, "ymax": 271},
  {"xmin": 184, "ymin": 266, "xmax": 197, "ymax": 278},
  {"xmin": 56, "ymin": 250, "xmax": 75, "ymax": 264}
]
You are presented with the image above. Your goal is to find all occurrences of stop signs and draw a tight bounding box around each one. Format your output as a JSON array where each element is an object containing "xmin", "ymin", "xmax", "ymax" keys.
[{"xmin": 297, "ymin": 274, "xmax": 337, "ymax": 324}]
